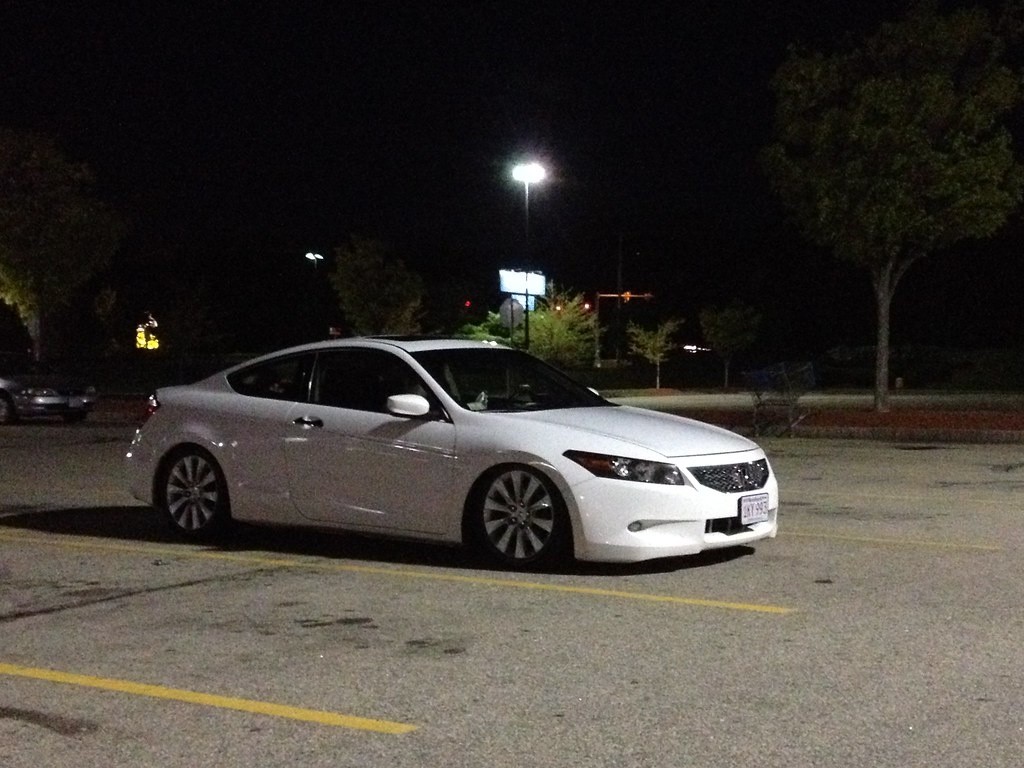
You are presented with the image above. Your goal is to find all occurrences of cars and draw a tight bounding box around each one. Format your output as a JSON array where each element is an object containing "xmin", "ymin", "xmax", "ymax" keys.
[
  {"xmin": 1, "ymin": 351, "xmax": 100, "ymax": 426},
  {"xmin": 125, "ymin": 337, "xmax": 779, "ymax": 571}
]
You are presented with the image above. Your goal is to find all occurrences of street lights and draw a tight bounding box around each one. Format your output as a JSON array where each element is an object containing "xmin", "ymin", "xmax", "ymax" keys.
[
  {"xmin": 304, "ymin": 252, "xmax": 324, "ymax": 270},
  {"xmin": 508, "ymin": 160, "xmax": 550, "ymax": 348}
]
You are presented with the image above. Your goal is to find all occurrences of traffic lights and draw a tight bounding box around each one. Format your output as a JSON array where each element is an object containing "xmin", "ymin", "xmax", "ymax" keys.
[{"xmin": 623, "ymin": 291, "xmax": 631, "ymax": 303}]
